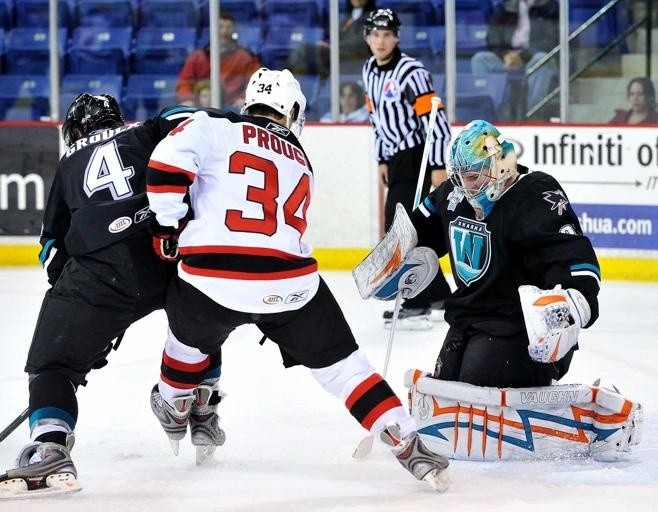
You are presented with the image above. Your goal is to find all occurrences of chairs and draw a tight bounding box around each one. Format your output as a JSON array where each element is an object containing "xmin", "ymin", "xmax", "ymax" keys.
[
  {"xmin": 314, "ymin": 75, "xmax": 368, "ymax": 121},
  {"xmin": 124, "ymin": 74, "xmax": 184, "ymax": 122},
  {"xmin": 1, "ymin": 74, "xmax": 59, "ymax": 121},
  {"xmin": 1, "ymin": 0, "xmax": 628, "ymax": 74},
  {"xmin": 438, "ymin": 75, "xmax": 509, "ymax": 121},
  {"xmin": 292, "ymin": 76, "xmax": 325, "ymax": 121},
  {"xmin": 59, "ymin": 74, "xmax": 125, "ymax": 122}
]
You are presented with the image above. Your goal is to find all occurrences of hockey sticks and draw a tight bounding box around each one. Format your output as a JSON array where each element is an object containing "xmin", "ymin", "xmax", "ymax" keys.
[{"xmin": 351, "ymin": 96, "xmax": 442, "ymax": 459}]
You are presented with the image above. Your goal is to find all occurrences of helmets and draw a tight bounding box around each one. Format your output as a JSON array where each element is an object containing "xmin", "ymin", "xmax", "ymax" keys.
[
  {"xmin": 447, "ymin": 119, "xmax": 520, "ymax": 222},
  {"xmin": 243, "ymin": 66, "xmax": 308, "ymax": 138},
  {"xmin": 363, "ymin": 9, "xmax": 402, "ymax": 38},
  {"xmin": 61, "ymin": 92, "xmax": 125, "ymax": 147}
]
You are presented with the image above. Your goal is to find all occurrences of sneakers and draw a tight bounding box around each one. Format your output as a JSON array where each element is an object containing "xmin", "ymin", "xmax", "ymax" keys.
[
  {"xmin": 383, "ymin": 301, "xmax": 445, "ymax": 319},
  {"xmin": 191, "ymin": 384, "xmax": 225, "ymax": 446},
  {"xmin": 392, "ymin": 430, "xmax": 449, "ymax": 483},
  {"xmin": 150, "ymin": 384, "xmax": 196, "ymax": 440},
  {"xmin": 0, "ymin": 430, "xmax": 77, "ymax": 490}
]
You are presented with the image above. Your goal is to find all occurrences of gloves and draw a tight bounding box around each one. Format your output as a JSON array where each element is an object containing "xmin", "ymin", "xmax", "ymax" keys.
[{"xmin": 149, "ymin": 214, "xmax": 190, "ymax": 263}]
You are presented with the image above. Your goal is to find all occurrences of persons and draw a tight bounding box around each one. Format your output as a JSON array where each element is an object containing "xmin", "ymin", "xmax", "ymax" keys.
[
  {"xmin": 360, "ymin": 8, "xmax": 452, "ymax": 323},
  {"xmin": 286, "ymin": 1, "xmax": 378, "ymax": 124},
  {"xmin": 350, "ymin": 119, "xmax": 601, "ymax": 389},
  {"xmin": 175, "ymin": 13, "xmax": 262, "ymax": 114},
  {"xmin": 144, "ymin": 65, "xmax": 450, "ymax": 483},
  {"xmin": 608, "ymin": 78, "xmax": 658, "ymax": 125},
  {"xmin": 0, "ymin": 92, "xmax": 226, "ymax": 492},
  {"xmin": 471, "ymin": 1, "xmax": 561, "ymax": 123}
]
